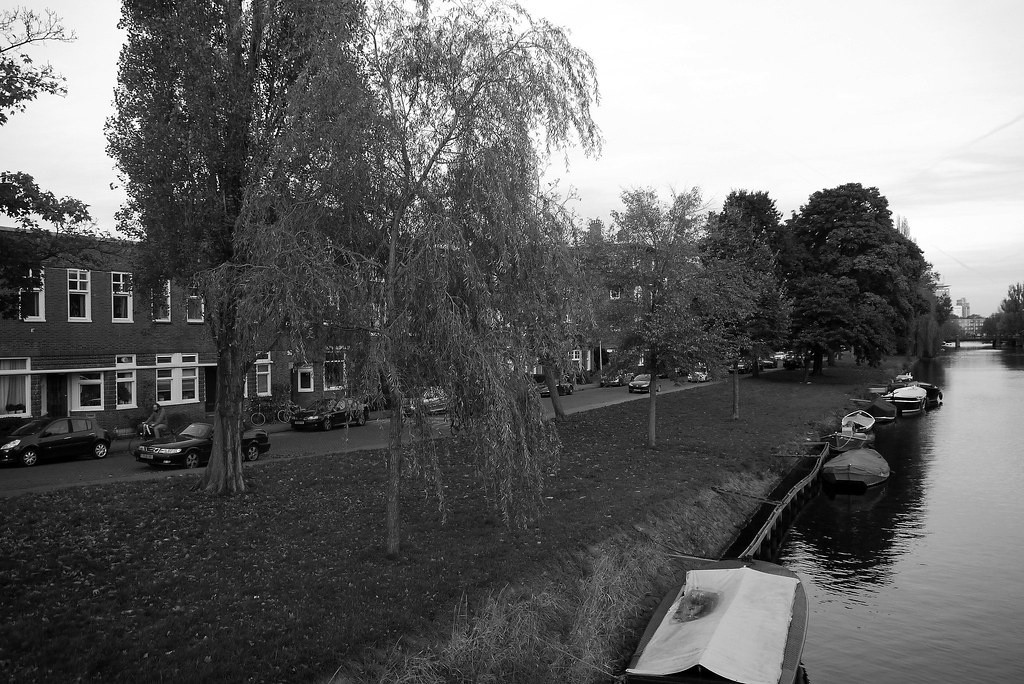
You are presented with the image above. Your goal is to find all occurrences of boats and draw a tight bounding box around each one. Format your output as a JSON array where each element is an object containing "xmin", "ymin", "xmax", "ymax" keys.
[
  {"xmin": 883, "ymin": 386, "xmax": 927, "ymax": 411},
  {"xmin": 860, "ymin": 397, "xmax": 897, "ymax": 422},
  {"xmin": 822, "ymin": 448, "xmax": 890, "ymax": 491},
  {"xmin": 827, "ymin": 432, "xmax": 875, "ymax": 452},
  {"xmin": 866, "ymin": 372, "xmax": 943, "ymax": 405},
  {"xmin": 841, "ymin": 409, "xmax": 876, "ymax": 433},
  {"xmin": 626, "ymin": 558, "xmax": 811, "ymax": 684}
]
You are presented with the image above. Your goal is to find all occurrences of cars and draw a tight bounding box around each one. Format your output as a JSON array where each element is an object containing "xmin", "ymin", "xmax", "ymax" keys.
[
  {"xmin": 628, "ymin": 373, "xmax": 662, "ymax": 393},
  {"xmin": 764, "ymin": 357, "xmax": 778, "ymax": 368},
  {"xmin": 774, "ymin": 351, "xmax": 787, "ymax": 360},
  {"xmin": 291, "ymin": 397, "xmax": 371, "ymax": 432},
  {"xmin": 687, "ymin": 366, "xmax": 713, "ymax": 382},
  {"xmin": 728, "ymin": 359, "xmax": 753, "ymax": 372},
  {"xmin": 402, "ymin": 387, "xmax": 453, "ymax": 417},
  {"xmin": 532, "ymin": 373, "xmax": 573, "ymax": 396},
  {"xmin": 133, "ymin": 422, "xmax": 271, "ymax": 468},
  {"xmin": 758, "ymin": 358, "xmax": 765, "ymax": 370},
  {"xmin": 600, "ymin": 371, "xmax": 634, "ymax": 388},
  {"xmin": 0, "ymin": 416, "xmax": 116, "ymax": 467}
]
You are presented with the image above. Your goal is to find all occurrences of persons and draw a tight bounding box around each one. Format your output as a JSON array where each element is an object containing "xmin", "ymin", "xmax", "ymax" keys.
[
  {"xmin": 799, "ymin": 347, "xmax": 812, "ymax": 384},
  {"xmin": 142, "ymin": 403, "xmax": 168, "ymax": 438},
  {"xmin": 631, "ymin": 373, "xmax": 634, "ymax": 381}
]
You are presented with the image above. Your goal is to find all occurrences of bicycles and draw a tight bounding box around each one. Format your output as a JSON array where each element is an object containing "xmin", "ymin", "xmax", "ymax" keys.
[
  {"xmin": 250, "ymin": 399, "xmax": 306, "ymax": 426},
  {"xmin": 128, "ymin": 424, "xmax": 175, "ymax": 455}
]
